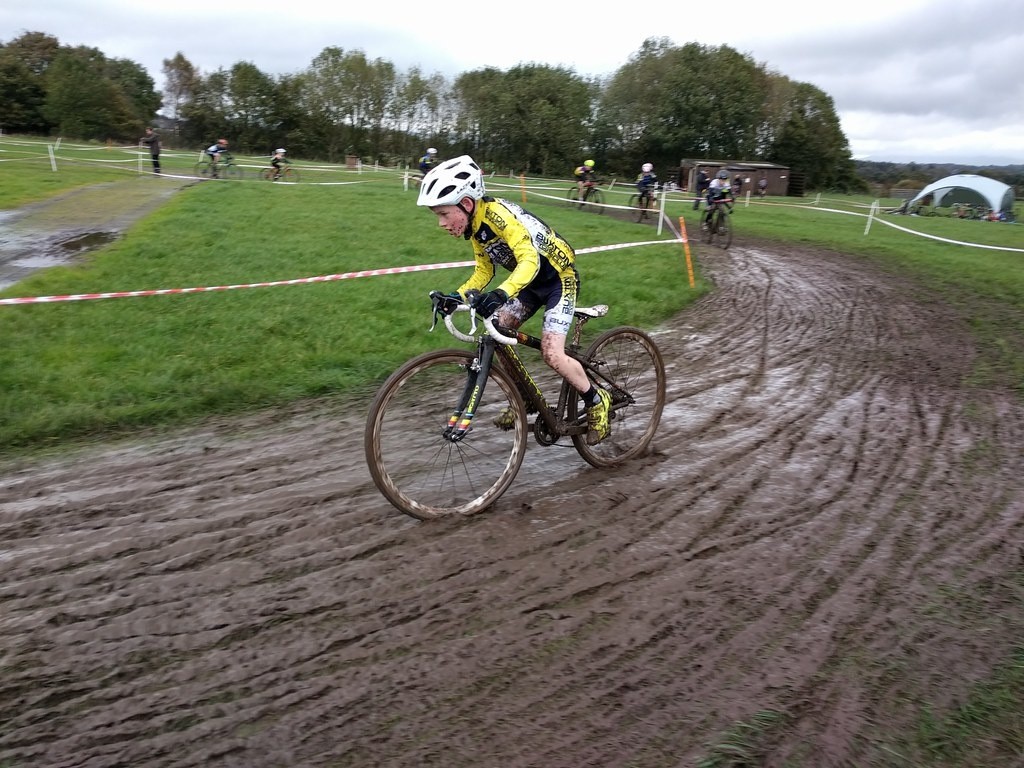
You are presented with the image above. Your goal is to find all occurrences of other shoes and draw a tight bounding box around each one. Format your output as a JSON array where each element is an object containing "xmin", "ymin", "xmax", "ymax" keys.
[{"xmin": 702, "ymin": 223, "xmax": 708, "ymax": 231}]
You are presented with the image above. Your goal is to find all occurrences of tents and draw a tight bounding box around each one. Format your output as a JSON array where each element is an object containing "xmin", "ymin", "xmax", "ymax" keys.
[{"xmin": 908, "ymin": 174, "xmax": 1015, "ymax": 215}]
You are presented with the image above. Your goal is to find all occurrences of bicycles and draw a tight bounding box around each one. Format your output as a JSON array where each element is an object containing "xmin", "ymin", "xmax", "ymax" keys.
[
  {"xmin": 700, "ymin": 197, "xmax": 735, "ymax": 250},
  {"xmin": 567, "ymin": 180, "xmax": 606, "ymax": 215},
  {"xmin": 628, "ymin": 185, "xmax": 661, "ymax": 223},
  {"xmin": 409, "ymin": 169, "xmax": 431, "ymax": 190},
  {"xmin": 363, "ymin": 288, "xmax": 667, "ymax": 519},
  {"xmin": 259, "ymin": 161, "xmax": 301, "ymax": 182},
  {"xmin": 193, "ymin": 156, "xmax": 243, "ymax": 182}
]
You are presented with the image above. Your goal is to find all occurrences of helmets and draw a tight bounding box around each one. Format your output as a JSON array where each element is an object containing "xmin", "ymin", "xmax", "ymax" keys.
[
  {"xmin": 642, "ymin": 163, "xmax": 653, "ymax": 172},
  {"xmin": 716, "ymin": 170, "xmax": 732, "ymax": 181},
  {"xmin": 585, "ymin": 160, "xmax": 594, "ymax": 166},
  {"xmin": 416, "ymin": 154, "xmax": 485, "ymax": 206},
  {"xmin": 427, "ymin": 147, "xmax": 437, "ymax": 154},
  {"xmin": 277, "ymin": 148, "xmax": 286, "ymax": 153},
  {"xmin": 219, "ymin": 139, "xmax": 229, "ymax": 145}
]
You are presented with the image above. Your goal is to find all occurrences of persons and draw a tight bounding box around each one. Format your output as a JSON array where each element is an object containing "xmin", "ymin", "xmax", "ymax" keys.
[
  {"xmin": 265, "ymin": 149, "xmax": 293, "ymax": 181},
  {"xmin": 634, "ymin": 162, "xmax": 658, "ymax": 208},
  {"xmin": 574, "ymin": 160, "xmax": 598, "ymax": 202},
  {"xmin": 416, "ymin": 155, "xmax": 614, "ymax": 446},
  {"xmin": 418, "ymin": 148, "xmax": 440, "ymax": 178},
  {"xmin": 957, "ymin": 206, "xmax": 1008, "ymax": 221},
  {"xmin": 669, "ymin": 169, "xmax": 769, "ymax": 234},
  {"xmin": 205, "ymin": 140, "xmax": 230, "ymax": 178},
  {"xmin": 139, "ymin": 127, "xmax": 160, "ymax": 173}
]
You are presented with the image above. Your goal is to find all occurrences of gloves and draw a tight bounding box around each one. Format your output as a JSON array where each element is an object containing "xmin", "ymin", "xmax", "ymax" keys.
[
  {"xmin": 431, "ymin": 292, "xmax": 463, "ymax": 319},
  {"xmin": 470, "ymin": 288, "xmax": 507, "ymax": 319}
]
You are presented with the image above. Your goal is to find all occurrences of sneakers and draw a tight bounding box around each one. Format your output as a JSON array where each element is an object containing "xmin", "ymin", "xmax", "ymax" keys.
[
  {"xmin": 491, "ymin": 402, "xmax": 531, "ymax": 425},
  {"xmin": 586, "ymin": 388, "xmax": 613, "ymax": 445}
]
